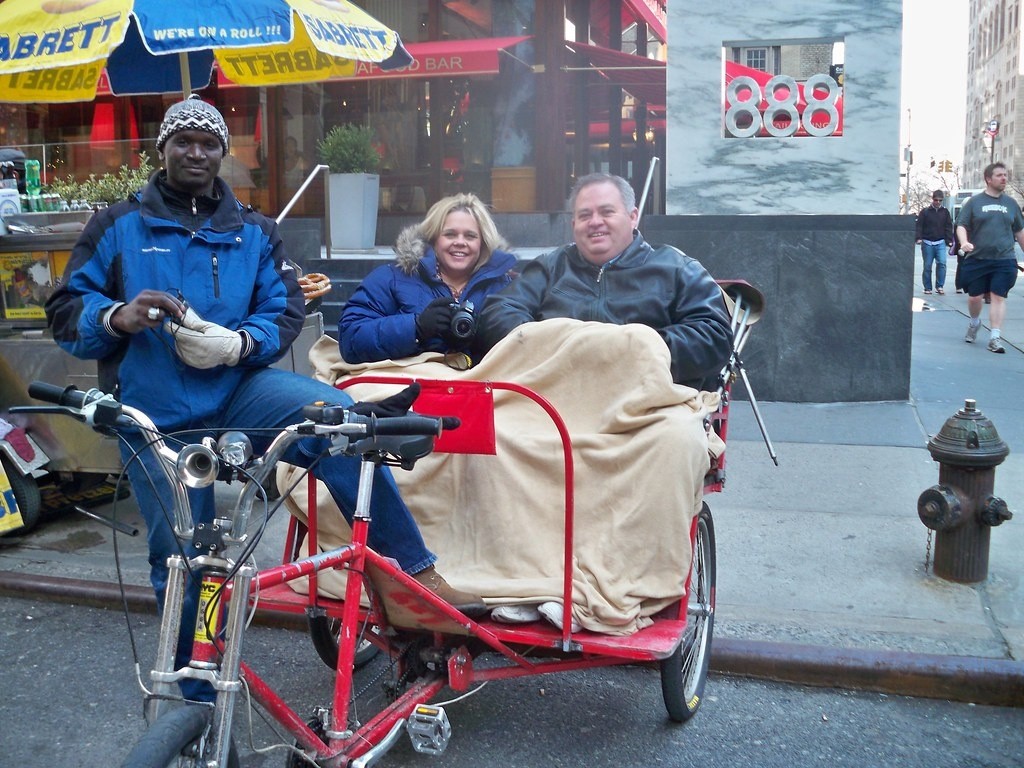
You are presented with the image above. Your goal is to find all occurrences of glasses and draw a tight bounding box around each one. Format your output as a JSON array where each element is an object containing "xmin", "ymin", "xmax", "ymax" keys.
[{"xmin": 934, "ymin": 199, "xmax": 942, "ymax": 202}]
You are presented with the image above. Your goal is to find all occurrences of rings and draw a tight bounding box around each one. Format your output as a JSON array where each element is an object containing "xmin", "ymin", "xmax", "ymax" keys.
[{"xmin": 148, "ymin": 305, "xmax": 160, "ymax": 321}]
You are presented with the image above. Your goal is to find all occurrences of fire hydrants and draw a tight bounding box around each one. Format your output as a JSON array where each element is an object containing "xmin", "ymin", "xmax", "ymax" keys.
[{"xmin": 917, "ymin": 398, "xmax": 1013, "ymax": 586}]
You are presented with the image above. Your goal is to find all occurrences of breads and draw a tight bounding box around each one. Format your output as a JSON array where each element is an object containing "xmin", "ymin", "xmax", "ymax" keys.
[{"xmin": 297, "ymin": 272, "xmax": 332, "ymax": 298}]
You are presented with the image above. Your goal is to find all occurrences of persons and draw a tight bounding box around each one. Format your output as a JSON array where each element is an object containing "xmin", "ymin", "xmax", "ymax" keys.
[
  {"xmin": 338, "ymin": 191, "xmax": 520, "ymax": 372},
  {"xmin": 916, "ymin": 190, "xmax": 953, "ymax": 295},
  {"xmin": 473, "ymin": 172, "xmax": 734, "ymax": 633},
  {"xmin": 43, "ymin": 92, "xmax": 453, "ymax": 756},
  {"xmin": 956, "ymin": 161, "xmax": 1024, "ymax": 352}
]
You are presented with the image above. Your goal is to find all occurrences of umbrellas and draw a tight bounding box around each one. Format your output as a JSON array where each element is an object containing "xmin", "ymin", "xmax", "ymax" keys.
[{"xmin": 0, "ymin": 0, "xmax": 415, "ymax": 101}]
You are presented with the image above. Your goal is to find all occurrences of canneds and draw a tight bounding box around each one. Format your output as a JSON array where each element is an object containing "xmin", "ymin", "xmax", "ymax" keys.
[{"xmin": 19, "ymin": 193, "xmax": 61, "ymax": 213}]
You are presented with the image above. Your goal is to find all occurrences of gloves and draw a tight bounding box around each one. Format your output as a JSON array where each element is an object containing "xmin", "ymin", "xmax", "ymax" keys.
[
  {"xmin": 352, "ymin": 383, "xmax": 460, "ymax": 470},
  {"xmin": 164, "ymin": 307, "xmax": 242, "ymax": 369},
  {"xmin": 416, "ymin": 298, "xmax": 455, "ymax": 343}
]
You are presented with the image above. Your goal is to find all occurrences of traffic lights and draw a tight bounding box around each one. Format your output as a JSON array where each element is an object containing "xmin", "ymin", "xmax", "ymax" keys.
[
  {"xmin": 938, "ymin": 160, "xmax": 944, "ymax": 173},
  {"xmin": 946, "ymin": 160, "xmax": 953, "ymax": 172}
]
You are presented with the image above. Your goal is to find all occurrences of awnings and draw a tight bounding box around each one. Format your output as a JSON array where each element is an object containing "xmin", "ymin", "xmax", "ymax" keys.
[
  {"xmin": 217, "ymin": 35, "xmax": 540, "ymax": 91},
  {"xmin": 568, "ymin": 39, "xmax": 667, "ymax": 105}
]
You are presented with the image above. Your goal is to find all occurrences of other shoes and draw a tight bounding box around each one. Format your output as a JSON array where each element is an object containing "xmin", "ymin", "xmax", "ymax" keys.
[
  {"xmin": 411, "ymin": 565, "xmax": 487, "ymax": 618},
  {"xmin": 956, "ymin": 288, "xmax": 961, "ymax": 293},
  {"xmin": 985, "ymin": 293, "xmax": 991, "ymax": 303},
  {"xmin": 924, "ymin": 289, "xmax": 933, "ymax": 294},
  {"xmin": 936, "ymin": 287, "xmax": 944, "ymax": 294}
]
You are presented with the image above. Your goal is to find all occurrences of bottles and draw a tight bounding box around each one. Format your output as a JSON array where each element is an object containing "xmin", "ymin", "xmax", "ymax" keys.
[{"xmin": 0, "ymin": 159, "xmax": 109, "ymax": 215}]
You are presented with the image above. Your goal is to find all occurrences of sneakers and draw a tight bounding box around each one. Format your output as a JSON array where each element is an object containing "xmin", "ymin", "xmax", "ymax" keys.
[
  {"xmin": 966, "ymin": 319, "xmax": 981, "ymax": 342},
  {"xmin": 491, "ymin": 605, "xmax": 539, "ymax": 623},
  {"xmin": 987, "ymin": 337, "xmax": 1005, "ymax": 353},
  {"xmin": 538, "ymin": 601, "xmax": 584, "ymax": 633}
]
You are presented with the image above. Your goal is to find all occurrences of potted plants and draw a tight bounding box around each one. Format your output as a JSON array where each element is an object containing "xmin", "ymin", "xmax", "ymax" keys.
[{"xmin": 314, "ymin": 122, "xmax": 384, "ymax": 252}]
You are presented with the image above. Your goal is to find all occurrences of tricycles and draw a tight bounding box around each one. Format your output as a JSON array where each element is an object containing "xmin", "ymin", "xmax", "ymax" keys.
[{"xmin": 6, "ymin": 267, "xmax": 765, "ymax": 768}]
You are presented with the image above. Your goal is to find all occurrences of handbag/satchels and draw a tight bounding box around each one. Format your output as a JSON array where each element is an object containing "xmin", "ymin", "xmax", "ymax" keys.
[{"xmin": 948, "ymin": 234, "xmax": 958, "ymax": 255}]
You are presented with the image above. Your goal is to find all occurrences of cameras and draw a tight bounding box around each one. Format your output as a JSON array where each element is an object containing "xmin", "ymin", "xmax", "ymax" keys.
[{"xmin": 448, "ymin": 300, "xmax": 480, "ymax": 339}]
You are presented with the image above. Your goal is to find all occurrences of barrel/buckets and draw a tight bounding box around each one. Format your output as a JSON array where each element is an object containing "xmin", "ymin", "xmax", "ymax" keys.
[{"xmin": 488, "ymin": 166, "xmax": 538, "ymax": 212}]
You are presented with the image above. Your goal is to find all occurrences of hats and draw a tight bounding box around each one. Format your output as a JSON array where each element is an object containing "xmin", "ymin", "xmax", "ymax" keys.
[
  {"xmin": 0, "ymin": 149, "xmax": 27, "ymax": 163},
  {"xmin": 156, "ymin": 94, "xmax": 229, "ymax": 157}
]
